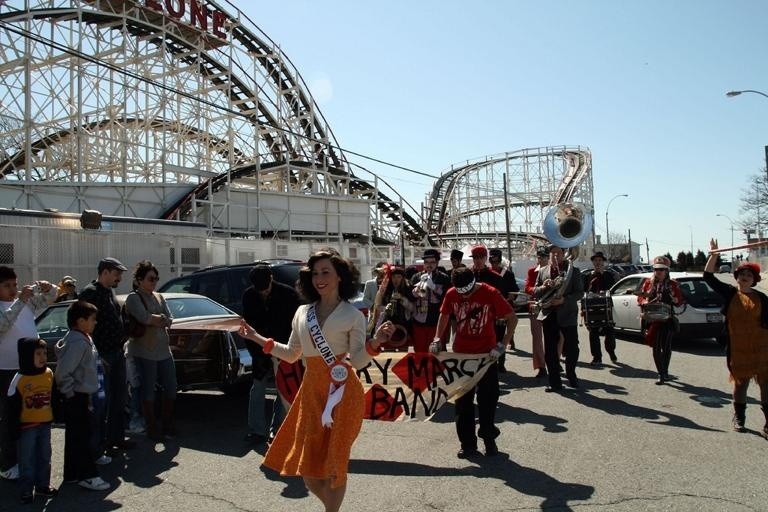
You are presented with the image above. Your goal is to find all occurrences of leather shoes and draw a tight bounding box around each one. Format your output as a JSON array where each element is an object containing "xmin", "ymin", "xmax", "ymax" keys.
[
  {"xmin": 656, "ymin": 376, "xmax": 664, "ymax": 384},
  {"xmin": 568, "ymin": 377, "xmax": 578, "ymax": 387},
  {"xmin": 545, "ymin": 382, "xmax": 562, "ymax": 392}
]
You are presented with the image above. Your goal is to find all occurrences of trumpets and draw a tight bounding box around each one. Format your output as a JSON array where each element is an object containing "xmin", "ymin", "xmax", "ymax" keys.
[{"xmin": 418, "ymin": 270, "xmax": 432, "ymax": 297}]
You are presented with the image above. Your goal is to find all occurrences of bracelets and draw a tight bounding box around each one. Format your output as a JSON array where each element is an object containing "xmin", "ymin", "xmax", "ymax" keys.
[
  {"xmin": 263, "ymin": 339, "xmax": 274, "ymax": 354},
  {"xmin": 366, "ymin": 340, "xmax": 381, "ymax": 356}
]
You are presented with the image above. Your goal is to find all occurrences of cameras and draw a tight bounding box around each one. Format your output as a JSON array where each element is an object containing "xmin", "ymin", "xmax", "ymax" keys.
[{"xmin": 30, "ymin": 284, "xmax": 42, "ymax": 293}]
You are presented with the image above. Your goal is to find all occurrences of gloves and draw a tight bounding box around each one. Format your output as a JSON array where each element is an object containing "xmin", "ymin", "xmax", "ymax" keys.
[
  {"xmin": 488, "ymin": 343, "xmax": 506, "ymax": 361},
  {"xmin": 428, "ymin": 338, "xmax": 442, "ymax": 354}
]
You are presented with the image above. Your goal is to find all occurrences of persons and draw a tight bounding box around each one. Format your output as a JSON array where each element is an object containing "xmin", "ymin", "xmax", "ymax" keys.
[
  {"xmin": 364, "ymin": 245, "xmax": 520, "ymax": 374},
  {"xmin": 125, "ymin": 261, "xmax": 179, "ymax": 465},
  {"xmin": 6, "ymin": 337, "xmax": 60, "ymax": 504},
  {"xmin": 638, "ymin": 256, "xmax": 683, "ymax": 384},
  {"xmin": 525, "ymin": 244, "xmax": 618, "ymax": 392},
  {"xmin": 81, "ymin": 257, "xmax": 137, "ymax": 458},
  {"xmin": 430, "ymin": 268, "xmax": 518, "ymax": 460},
  {"xmin": 53, "ymin": 302, "xmax": 110, "ymax": 491},
  {"xmin": 703, "ymin": 239, "xmax": 768, "ymax": 438},
  {"xmin": 239, "ymin": 243, "xmax": 393, "ymax": 512},
  {"xmin": 1, "ymin": 265, "xmax": 59, "ymax": 480},
  {"xmin": 57, "ymin": 276, "xmax": 80, "ymax": 302},
  {"xmin": 89, "ymin": 359, "xmax": 113, "ymax": 465},
  {"xmin": 123, "ymin": 339, "xmax": 145, "ymax": 431},
  {"xmin": 239, "ymin": 263, "xmax": 304, "ymax": 456}
]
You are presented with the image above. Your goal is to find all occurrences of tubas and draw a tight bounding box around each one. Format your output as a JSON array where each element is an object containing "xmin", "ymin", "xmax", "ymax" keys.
[{"xmin": 528, "ymin": 202, "xmax": 592, "ymax": 310}]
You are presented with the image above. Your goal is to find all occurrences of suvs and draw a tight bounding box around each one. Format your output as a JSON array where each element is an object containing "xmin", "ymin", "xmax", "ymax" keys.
[{"xmin": 156, "ymin": 259, "xmax": 313, "ymax": 353}]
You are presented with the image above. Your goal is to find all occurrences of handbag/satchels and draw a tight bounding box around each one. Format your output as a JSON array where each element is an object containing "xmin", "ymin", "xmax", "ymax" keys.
[
  {"xmin": 52, "ymin": 384, "xmax": 106, "ymax": 425},
  {"xmin": 121, "ymin": 291, "xmax": 148, "ymax": 338}
]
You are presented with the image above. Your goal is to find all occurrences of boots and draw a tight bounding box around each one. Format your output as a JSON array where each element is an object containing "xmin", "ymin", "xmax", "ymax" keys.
[{"xmin": 733, "ymin": 402, "xmax": 746, "ymax": 433}]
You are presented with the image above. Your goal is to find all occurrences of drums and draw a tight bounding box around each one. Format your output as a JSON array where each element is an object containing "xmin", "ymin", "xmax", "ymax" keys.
[
  {"xmin": 644, "ymin": 303, "xmax": 671, "ymax": 319},
  {"xmin": 583, "ymin": 290, "xmax": 614, "ymax": 327}
]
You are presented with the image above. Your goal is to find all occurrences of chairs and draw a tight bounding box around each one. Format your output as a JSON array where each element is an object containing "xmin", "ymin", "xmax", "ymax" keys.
[
  {"xmin": 679, "ymin": 284, "xmax": 690, "ymax": 293},
  {"xmin": 695, "ymin": 284, "xmax": 708, "ymax": 297}
]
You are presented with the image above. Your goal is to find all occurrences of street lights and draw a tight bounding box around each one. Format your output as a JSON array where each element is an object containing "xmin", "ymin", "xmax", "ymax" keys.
[
  {"xmin": 715, "ymin": 213, "xmax": 735, "ymax": 269},
  {"xmin": 726, "ymin": 90, "xmax": 768, "ymax": 102},
  {"xmin": 605, "ymin": 193, "xmax": 628, "ymax": 256}
]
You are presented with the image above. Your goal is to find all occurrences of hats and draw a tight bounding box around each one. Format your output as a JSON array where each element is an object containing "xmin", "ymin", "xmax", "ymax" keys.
[
  {"xmin": 62, "ymin": 275, "xmax": 74, "ymax": 285},
  {"xmin": 652, "ymin": 256, "xmax": 672, "ymax": 272},
  {"xmin": 422, "ymin": 249, "xmax": 441, "ymax": 260},
  {"xmin": 99, "ymin": 257, "xmax": 128, "ymax": 275},
  {"xmin": 451, "ymin": 250, "xmax": 464, "ymax": 260},
  {"xmin": 733, "ymin": 263, "xmax": 761, "ymax": 285},
  {"xmin": 249, "ymin": 264, "xmax": 272, "ymax": 291},
  {"xmin": 591, "ymin": 252, "xmax": 608, "ymax": 263},
  {"xmin": 538, "ymin": 246, "xmax": 549, "ymax": 257},
  {"xmin": 490, "ymin": 250, "xmax": 502, "ymax": 258},
  {"xmin": 471, "ymin": 247, "xmax": 487, "ymax": 257}
]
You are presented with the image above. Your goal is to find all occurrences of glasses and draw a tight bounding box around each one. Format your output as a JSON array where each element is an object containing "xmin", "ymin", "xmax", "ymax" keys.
[{"xmin": 149, "ymin": 276, "xmax": 159, "ymax": 282}]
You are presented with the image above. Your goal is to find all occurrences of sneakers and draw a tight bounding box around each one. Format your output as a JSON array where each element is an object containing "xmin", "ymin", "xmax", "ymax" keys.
[
  {"xmin": 458, "ymin": 443, "xmax": 478, "ymax": 459},
  {"xmin": 19, "ymin": 491, "xmax": 33, "ymax": 505},
  {"xmin": 592, "ymin": 358, "xmax": 602, "ymax": 365},
  {"xmin": 79, "ymin": 475, "xmax": 111, "ymax": 491},
  {"xmin": 35, "ymin": 486, "xmax": 57, "ymax": 497},
  {"xmin": 485, "ymin": 439, "xmax": 497, "ymax": 457},
  {"xmin": 1, "ymin": 463, "xmax": 19, "ymax": 481},
  {"xmin": 95, "ymin": 455, "xmax": 113, "ymax": 465},
  {"xmin": 244, "ymin": 432, "xmax": 268, "ymax": 442}
]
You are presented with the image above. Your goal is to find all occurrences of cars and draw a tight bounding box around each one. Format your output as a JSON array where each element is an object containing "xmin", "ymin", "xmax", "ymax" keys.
[
  {"xmin": 508, "ymin": 277, "xmax": 532, "ymax": 312},
  {"xmin": 31, "ymin": 293, "xmax": 267, "ymax": 415},
  {"xmin": 718, "ymin": 264, "xmax": 730, "ymax": 273},
  {"xmin": 352, "ymin": 291, "xmax": 370, "ymax": 339},
  {"xmin": 577, "ymin": 264, "xmax": 663, "ymax": 287},
  {"xmin": 607, "ymin": 271, "xmax": 726, "ymax": 344}
]
